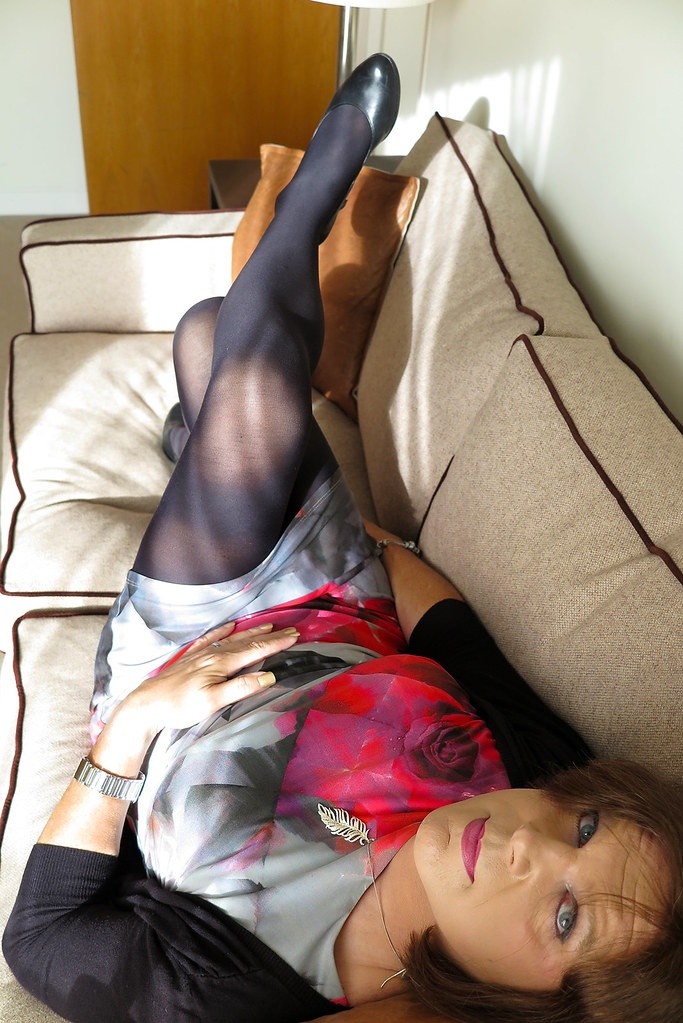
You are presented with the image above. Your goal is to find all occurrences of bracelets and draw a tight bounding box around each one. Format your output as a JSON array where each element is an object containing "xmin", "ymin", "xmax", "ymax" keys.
[
  {"xmin": 74, "ymin": 756, "xmax": 146, "ymax": 804},
  {"xmin": 374, "ymin": 537, "xmax": 423, "ymax": 560}
]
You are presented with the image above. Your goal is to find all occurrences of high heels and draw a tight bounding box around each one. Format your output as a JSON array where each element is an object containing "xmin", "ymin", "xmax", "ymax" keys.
[
  {"xmin": 272, "ymin": 52, "xmax": 399, "ymax": 246},
  {"xmin": 161, "ymin": 402, "xmax": 186, "ymax": 464}
]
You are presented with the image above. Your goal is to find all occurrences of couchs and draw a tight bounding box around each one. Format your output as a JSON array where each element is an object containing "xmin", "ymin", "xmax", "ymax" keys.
[{"xmin": 0, "ymin": 113, "xmax": 682, "ymax": 1023}]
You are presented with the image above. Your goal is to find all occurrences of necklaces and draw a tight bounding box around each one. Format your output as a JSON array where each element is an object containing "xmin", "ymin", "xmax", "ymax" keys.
[{"xmin": 315, "ymin": 804, "xmax": 440, "ymax": 992}]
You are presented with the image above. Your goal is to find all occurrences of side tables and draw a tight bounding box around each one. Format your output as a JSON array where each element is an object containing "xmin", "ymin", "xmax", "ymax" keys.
[{"xmin": 206, "ymin": 158, "xmax": 263, "ymax": 210}]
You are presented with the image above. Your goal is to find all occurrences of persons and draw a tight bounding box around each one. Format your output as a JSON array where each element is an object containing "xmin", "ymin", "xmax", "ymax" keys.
[{"xmin": 2, "ymin": 51, "xmax": 681, "ymax": 1022}]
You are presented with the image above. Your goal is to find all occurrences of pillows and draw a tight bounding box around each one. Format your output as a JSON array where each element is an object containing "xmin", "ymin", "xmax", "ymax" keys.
[{"xmin": 227, "ymin": 139, "xmax": 419, "ymax": 423}]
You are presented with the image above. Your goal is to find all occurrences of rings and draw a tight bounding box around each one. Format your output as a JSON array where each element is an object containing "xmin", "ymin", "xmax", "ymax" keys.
[{"xmin": 211, "ymin": 641, "xmax": 222, "ymax": 650}]
